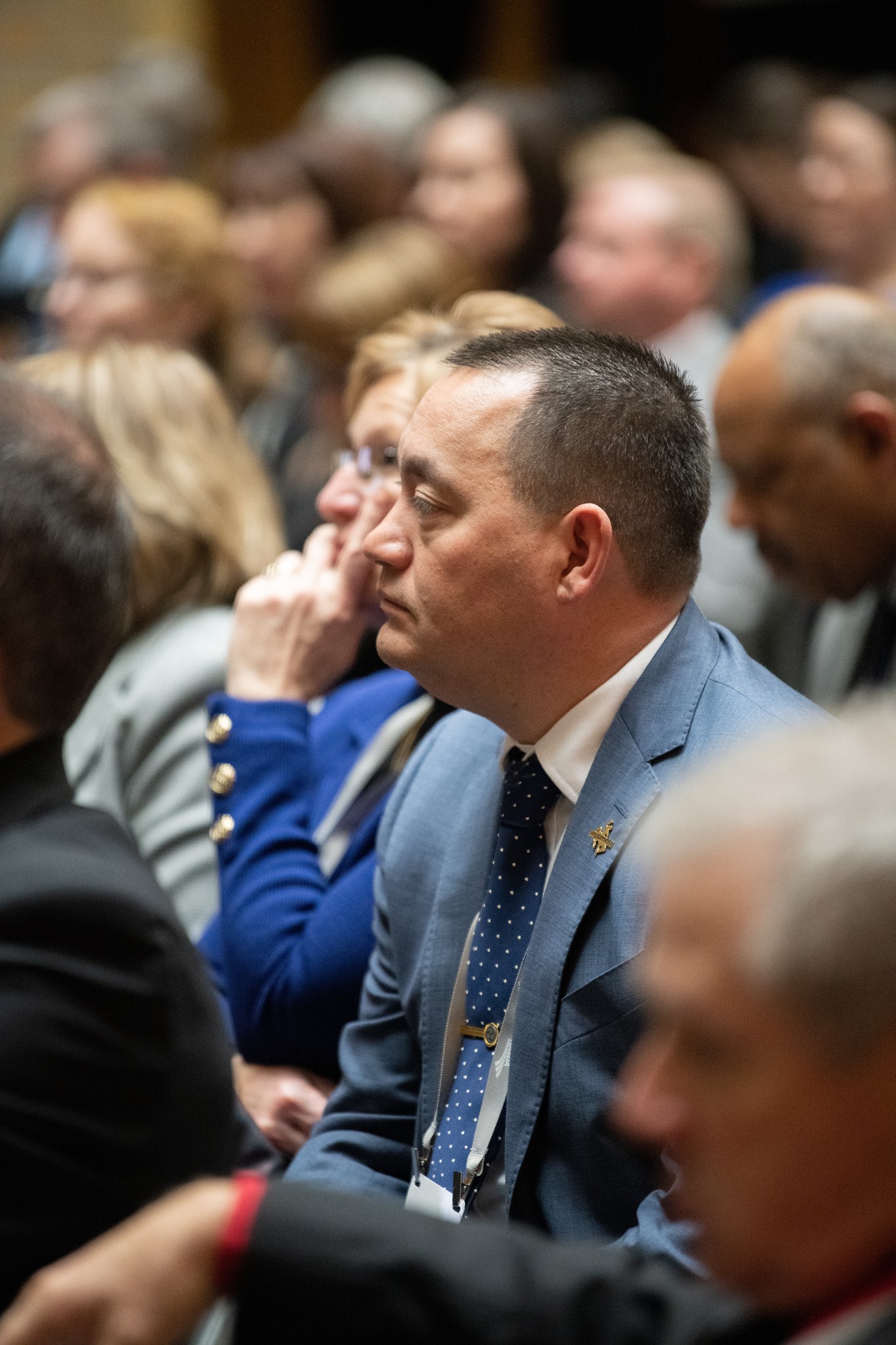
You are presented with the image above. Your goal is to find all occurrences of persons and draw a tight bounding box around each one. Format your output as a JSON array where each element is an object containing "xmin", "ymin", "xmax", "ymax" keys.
[
  {"xmin": 303, "ymin": 56, "xmax": 453, "ymax": 224},
  {"xmin": 281, "ymin": 324, "xmax": 835, "ymax": 1280},
  {"xmin": 11, "ymin": 342, "xmax": 285, "ymax": 941},
  {"xmin": 228, "ymin": 141, "xmax": 473, "ymax": 551},
  {"xmin": 0, "ymin": 61, "xmax": 212, "ymax": 360},
  {"xmin": 714, "ymin": 285, "xmax": 896, "ymax": 708},
  {"xmin": 401, "ymin": 85, "xmax": 569, "ymax": 304},
  {"xmin": 45, "ymin": 171, "xmax": 309, "ymax": 464},
  {"xmin": 0, "ymin": 371, "xmax": 235, "ymax": 1316},
  {"xmin": 0, "ymin": 694, "xmax": 896, "ymax": 1345},
  {"xmin": 551, "ymin": 149, "xmax": 772, "ymax": 638},
  {"xmin": 188, "ymin": 292, "xmax": 565, "ymax": 1152},
  {"xmin": 735, "ymin": 78, "xmax": 896, "ymax": 325}
]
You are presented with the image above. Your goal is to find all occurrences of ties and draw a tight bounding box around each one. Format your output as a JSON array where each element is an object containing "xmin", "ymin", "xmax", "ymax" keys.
[{"xmin": 426, "ymin": 745, "xmax": 565, "ymax": 1195}]
